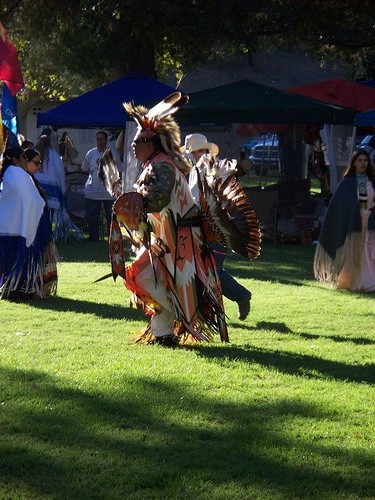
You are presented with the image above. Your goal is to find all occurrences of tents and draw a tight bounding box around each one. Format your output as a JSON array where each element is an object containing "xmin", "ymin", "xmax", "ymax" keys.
[{"xmin": 37, "ymin": 69, "xmax": 375, "ymax": 195}]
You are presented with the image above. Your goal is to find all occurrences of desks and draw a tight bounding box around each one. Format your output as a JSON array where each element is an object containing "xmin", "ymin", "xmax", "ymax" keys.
[{"xmin": 244, "ymin": 189, "xmax": 278, "ymax": 245}]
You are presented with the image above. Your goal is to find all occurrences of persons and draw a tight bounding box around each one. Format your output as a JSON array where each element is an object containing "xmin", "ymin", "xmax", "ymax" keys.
[
  {"xmin": 80, "ymin": 131, "xmax": 123, "ymax": 242},
  {"xmin": 359, "ymin": 135, "xmax": 375, "ymax": 169},
  {"xmin": 313, "ymin": 148, "xmax": 375, "ymax": 294},
  {"xmin": 306, "ymin": 138, "xmax": 330, "ymax": 173},
  {"xmin": 0, "ymin": 127, "xmax": 76, "ymax": 303},
  {"xmin": 108, "ymin": 93, "xmax": 262, "ymax": 347}
]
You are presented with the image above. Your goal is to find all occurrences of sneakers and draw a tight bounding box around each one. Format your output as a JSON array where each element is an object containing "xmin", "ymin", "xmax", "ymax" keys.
[{"xmin": 236, "ymin": 296, "xmax": 251, "ymax": 321}]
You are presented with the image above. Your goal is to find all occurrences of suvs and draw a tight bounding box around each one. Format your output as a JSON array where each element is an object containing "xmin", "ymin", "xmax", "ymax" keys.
[
  {"xmin": 249, "ymin": 141, "xmax": 282, "ymax": 177},
  {"xmin": 355, "ymin": 135, "xmax": 375, "ymax": 168}
]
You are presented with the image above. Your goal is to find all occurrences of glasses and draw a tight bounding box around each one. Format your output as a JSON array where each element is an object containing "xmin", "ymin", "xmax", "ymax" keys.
[
  {"xmin": 16, "ymin": 154, "xmax": 26, "ymax": 159},
  {"xmin": 32, "ymin": 159, "xmax": 42, "ymax": 166}
]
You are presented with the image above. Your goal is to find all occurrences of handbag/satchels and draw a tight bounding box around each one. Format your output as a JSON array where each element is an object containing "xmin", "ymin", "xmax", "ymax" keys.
[{"xmin": 59, "ymin": 132, "xmax": 79, "ymax": 160}]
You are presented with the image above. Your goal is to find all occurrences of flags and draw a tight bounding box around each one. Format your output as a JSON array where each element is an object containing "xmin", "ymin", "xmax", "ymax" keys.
[{"xmin": 0, "ymin": 22, "xmax": 24, "ymax": 148}]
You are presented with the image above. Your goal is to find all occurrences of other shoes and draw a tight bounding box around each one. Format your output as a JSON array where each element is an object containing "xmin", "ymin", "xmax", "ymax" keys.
[{"xmin": 153, "ymin": 335, "xmax": 179, "ymax": 346}]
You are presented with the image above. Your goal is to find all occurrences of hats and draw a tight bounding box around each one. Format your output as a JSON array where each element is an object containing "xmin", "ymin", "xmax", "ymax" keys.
[{"xmin": 180, "ymin": 134, "xmax": 219, "ymax": 156}]
